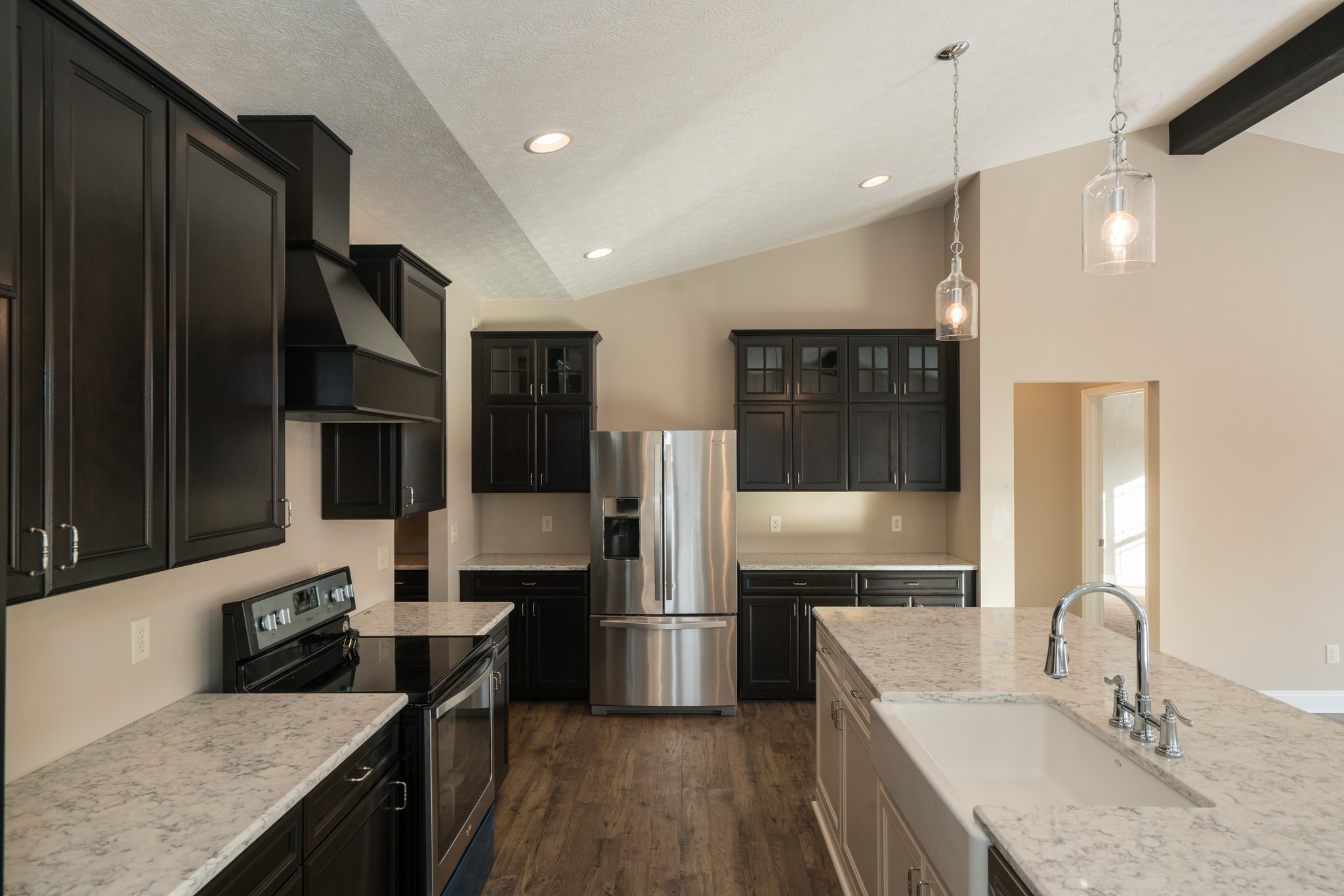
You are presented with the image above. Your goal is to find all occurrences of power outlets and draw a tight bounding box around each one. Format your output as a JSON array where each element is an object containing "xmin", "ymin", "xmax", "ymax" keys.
[
  {"xmin": 378, "ymin": 544, "xmax": 389, "ymax": 572},
  {"xmin": 451, "ymin": 523, "xmax": 458, "ymax": 545},
  {"xmin": 891, "ymin": 515, "xmax": 902, "ymax": 532},
  {"xmin": 1326, "ymin": 645, "xmax": 1340, "ymax": 664},
  {"xmin": 131, "ymin": 615, "xmax": 151, "ymax": 666},
  {"xmin": 317, "ymin": 561, "xmax": 326, "ymax": 573},
  {"xmin": 542, "ymin": 515, "xmax": 553, "ymax": 532},
  {"xmin": 769, "ymin": 515, "xmax": 780, "ymax": 532}
]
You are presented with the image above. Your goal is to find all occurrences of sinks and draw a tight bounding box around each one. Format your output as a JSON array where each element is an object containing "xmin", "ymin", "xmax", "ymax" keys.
[{"xmin": 879, "ymin": 688, "xmax": 1217, "ymax": 844}]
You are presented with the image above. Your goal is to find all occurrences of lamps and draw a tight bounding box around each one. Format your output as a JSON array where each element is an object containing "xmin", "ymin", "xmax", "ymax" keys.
[
  {"xmin": 935, "ymin": 37, "xmax": 982, "ymax": 341},
  {"xmin": 1084, "ymin": 0, "xmax": 1158, "ymax": 281}
]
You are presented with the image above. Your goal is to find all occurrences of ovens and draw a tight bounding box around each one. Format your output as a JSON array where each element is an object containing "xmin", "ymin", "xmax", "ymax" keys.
[{"xmin": 369, "ymin": 645, "xmax": 508, "ymax": 896}]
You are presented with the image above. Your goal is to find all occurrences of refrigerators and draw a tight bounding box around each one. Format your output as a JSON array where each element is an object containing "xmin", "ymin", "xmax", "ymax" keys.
[{"xmin": 590, "ymin": 427, "xmax": 741, "ymax": 718}]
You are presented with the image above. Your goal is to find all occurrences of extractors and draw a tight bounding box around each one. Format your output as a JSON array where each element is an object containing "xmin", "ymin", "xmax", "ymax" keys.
[{"xmin": 229, "ymin": 114, "xmax": 443, "ymax": 432}]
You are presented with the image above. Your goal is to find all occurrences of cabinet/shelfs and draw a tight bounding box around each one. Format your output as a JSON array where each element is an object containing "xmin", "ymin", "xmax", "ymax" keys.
[
  {"xmin": 485, "ymin": 615, "xmax": 510, "ymax": 799},
  {"xmin": 741, "ymin": 571, "xmax": 860, "ymax": 699},
  {"xmin": 849, "ymin": 327, "xmax": 961, "ymax": 494},
  {"xmin": 810, "ymin": 620, "xmax": 883, "ymax": 896},
  {"xmin": 1, "ymin": 0, "xmax": 288, "ymax": 612},
  {"xmin": 858, "ymin": 569, "xmax": 975, "ymax": 608},
  {"xmin": 873, "ymin": 780, "xmax": 950, "ymax": 896},
  {"xmin": 192, "ymin": 718, "xmax": 407, "ymax": 895},
  {"xmin": 728, "ymin": 327, "xmax": 849, "ymax": 495},
  {"xmin": 315, "ymin": 242, "xmax": 603, "ymax": 520},
  {"xmin": 464, "ymin": 571, "xmax": 588, "ymax": 701}
]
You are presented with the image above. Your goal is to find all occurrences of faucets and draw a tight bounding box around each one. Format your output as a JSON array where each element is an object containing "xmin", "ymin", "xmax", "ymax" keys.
[{"xmin": 1042, "ymin": 581, "xmax": 1163, "ymax": 747}]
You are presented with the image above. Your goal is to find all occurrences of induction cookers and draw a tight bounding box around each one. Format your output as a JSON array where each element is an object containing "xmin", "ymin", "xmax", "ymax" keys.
[{"xmin": 220, "ymin": 564, "xmax": 494, "ymax": 706}]
